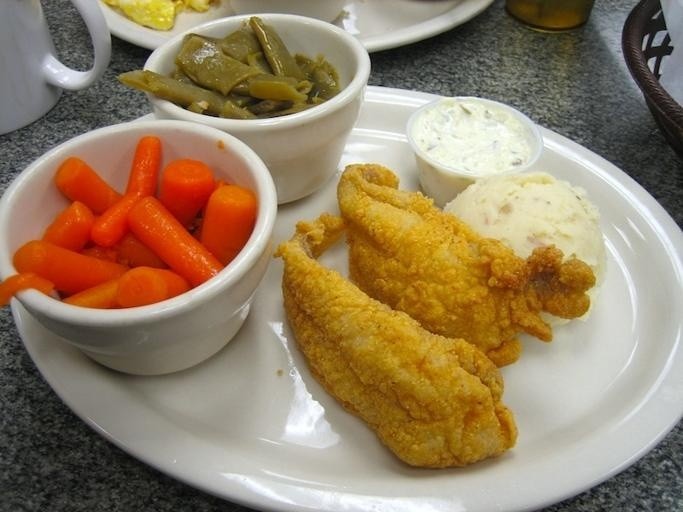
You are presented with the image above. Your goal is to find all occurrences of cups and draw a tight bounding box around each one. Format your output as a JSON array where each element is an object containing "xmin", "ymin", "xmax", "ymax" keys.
[{"xmin": 0, "ymin": 0, "xmax": 112, "ymax": 137}]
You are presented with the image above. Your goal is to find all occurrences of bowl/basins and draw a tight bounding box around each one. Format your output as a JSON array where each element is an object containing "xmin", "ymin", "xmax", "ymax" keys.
[
  {"xmin": 0, "ymin": 118, "xmax": 278, "ymax": 377},
  {"xmin": 143, "ymin": 13, "xmax": 373, "ymax": 204},
  {"xmin": 409, "ymin": 95, "xmax": 544, "ymax": 209}
]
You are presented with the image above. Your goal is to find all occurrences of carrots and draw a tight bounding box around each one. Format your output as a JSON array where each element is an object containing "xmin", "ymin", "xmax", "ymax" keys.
[{"xmin": 0, "ymin": 136, "xmax": 256, "ymax": 306}]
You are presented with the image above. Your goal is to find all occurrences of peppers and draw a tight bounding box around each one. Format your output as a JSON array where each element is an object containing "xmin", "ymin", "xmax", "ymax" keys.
[{"xmin": 115, "ymin": 17, "xmax": 342, "ymax": 119}]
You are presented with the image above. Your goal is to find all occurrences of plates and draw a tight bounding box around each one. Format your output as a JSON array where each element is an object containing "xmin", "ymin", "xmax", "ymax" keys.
[
  {"xmin": 99, "ymin": 0, "xmax": 497, "ymax": 56},
  {"xmin": 9, "ymin": 84, "xmax": 683, "ymax": 511}
]
